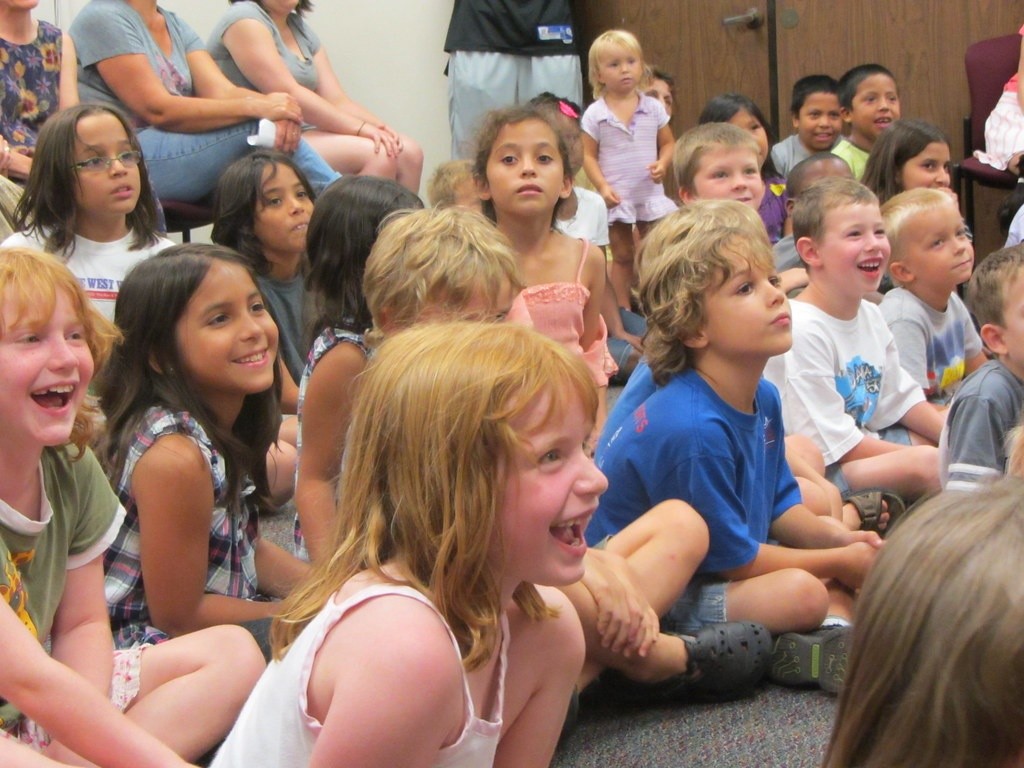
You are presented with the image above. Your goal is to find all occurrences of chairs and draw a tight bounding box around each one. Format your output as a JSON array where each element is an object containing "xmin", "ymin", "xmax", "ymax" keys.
[
  {"xmin": 153, "ymin": 194, "xmax": 217, "ymax": 246},
  {"xmin": 956, "ymin": 34, "xmax": 1024, "ymax": 250}
]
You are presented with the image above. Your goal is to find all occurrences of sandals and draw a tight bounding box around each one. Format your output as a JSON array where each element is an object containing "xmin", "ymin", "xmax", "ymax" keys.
[
  {"xmin": 648, "ymin": 620, "xmax": 772, "ymax": 703},
  {"xmin": 842, "ymin": 491, "xmax": 906, "ymax": 538}
]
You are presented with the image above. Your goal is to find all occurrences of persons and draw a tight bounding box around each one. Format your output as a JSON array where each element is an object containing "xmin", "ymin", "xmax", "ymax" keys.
[{"xmin": 0, "ymin": 0, "xmax": 1024, "ymax": 768}]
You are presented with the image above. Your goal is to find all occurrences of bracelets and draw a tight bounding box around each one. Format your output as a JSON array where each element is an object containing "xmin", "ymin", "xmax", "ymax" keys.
[{"xmin": 357, "ymin": 122, "xmax": 366, "ymax": 135}]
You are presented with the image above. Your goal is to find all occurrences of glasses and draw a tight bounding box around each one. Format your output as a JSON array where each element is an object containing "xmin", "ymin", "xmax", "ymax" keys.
[{"xmin": 69, "ymin": 151, "xmax": 142, "ymax": 173}]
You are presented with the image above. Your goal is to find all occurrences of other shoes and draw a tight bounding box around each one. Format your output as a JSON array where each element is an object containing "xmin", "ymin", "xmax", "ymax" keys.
[{"xmin": 764, "ymin": 624, "xmax": 854, "ymax": 693}]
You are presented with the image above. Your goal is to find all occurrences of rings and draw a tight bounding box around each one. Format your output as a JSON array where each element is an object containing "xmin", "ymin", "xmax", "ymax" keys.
[
  {"xmin": 5, "ymin": 147, "xmax": 11, "ymax": 152},
  {"xmin": 293, "ymin": 131, "xmax": 299, "ymax": 135}
]
